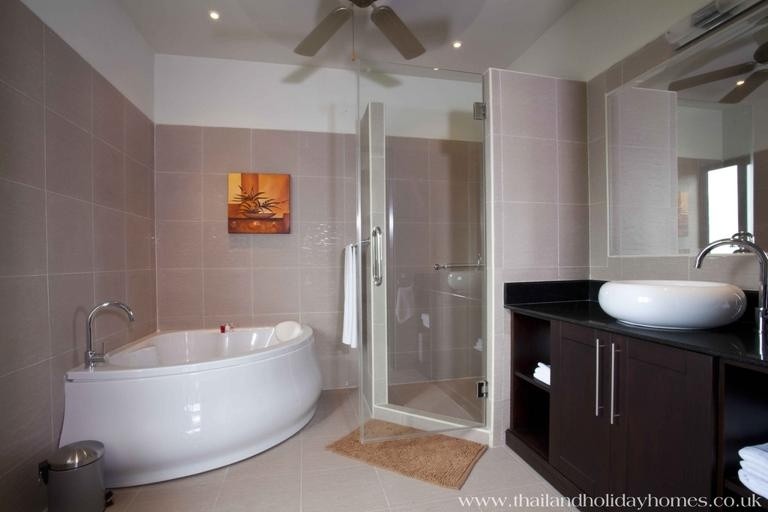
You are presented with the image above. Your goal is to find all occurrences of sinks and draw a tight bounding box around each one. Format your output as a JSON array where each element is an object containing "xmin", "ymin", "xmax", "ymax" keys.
[
  {"xmin": 448, "ymin": 268, "xmax": 482, "ymax": 290},
  {"xmin": 599, "ymin": 278, "xmax": 748, "ymax": 330}
]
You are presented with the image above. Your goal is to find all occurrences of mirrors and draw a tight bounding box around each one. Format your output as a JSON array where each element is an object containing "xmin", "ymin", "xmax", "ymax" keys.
[{"xmin": 603, "ymin": 1, "xmax": 768, "ymax": 260}]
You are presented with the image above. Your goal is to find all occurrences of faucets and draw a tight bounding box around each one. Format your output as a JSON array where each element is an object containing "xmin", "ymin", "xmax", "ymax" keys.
[
  {"xmin": 696, "ymin": 237, "xmax": 764, "ymax": 314},
  {"xmin": 83, "ymin": 300, "xmax": 134, "ymax": 366}
]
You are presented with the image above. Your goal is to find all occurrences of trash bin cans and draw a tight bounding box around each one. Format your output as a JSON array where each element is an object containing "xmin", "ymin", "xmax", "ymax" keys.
[{"xmin": 37, "ymin": 440, "xmax": 114, "ymax": 512}]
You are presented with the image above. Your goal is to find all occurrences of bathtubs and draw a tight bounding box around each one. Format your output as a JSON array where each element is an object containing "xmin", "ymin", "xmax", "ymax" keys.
[{"xmin": 61, "ymin": 319, "xmax": 322, "ymax": 490}]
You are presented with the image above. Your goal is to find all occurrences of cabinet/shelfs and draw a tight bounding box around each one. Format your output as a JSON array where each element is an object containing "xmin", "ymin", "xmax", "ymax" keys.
[
  {"xmin": 550, "ymin": 317, "xmax": 716, "ymax": 510},
  {"xmin": 509, "ymin": 303, "xmax": 549, "ymax": 481},
  {"xmin": 716, "ymin": 349, "xmax": 768, "ymax": 509}
]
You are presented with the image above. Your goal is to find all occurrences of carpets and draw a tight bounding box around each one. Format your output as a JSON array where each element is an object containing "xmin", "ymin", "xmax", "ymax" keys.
[{"xmin": 324, "ymin": 417, "xmax": 490, "ymax": 493}]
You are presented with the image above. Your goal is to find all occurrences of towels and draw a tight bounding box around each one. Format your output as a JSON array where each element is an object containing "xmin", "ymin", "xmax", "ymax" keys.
[
  {"xmin": 533, "ymin": 360, "xmax": 550, "ymax": 385},
  {"xmin": 737, "ymin": 443, "xmax": 768, "ymax": 500},
  {"xmin": 340, "ymin": 243, "xmax": 361, "ymax": 348}
]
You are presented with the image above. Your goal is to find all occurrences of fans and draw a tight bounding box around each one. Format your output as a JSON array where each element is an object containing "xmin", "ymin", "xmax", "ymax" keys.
[
  {"xmin": 290, "ymin": 0, "xmax": 426, "ymax": 63},
  {"xmin": 667, "ymin": 39, "xmax": 768, "ymax": 106}
]
